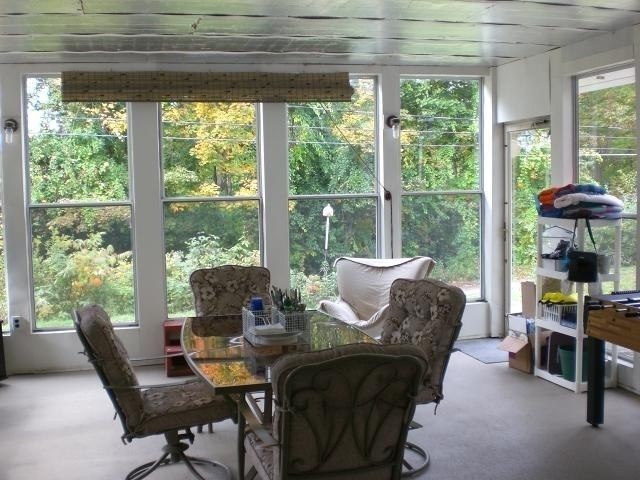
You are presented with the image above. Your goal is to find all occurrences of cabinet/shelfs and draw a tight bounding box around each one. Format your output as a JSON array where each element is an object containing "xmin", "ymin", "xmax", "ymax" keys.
[
  {"xmin": 532, "ymin": 216, "xmax": 622, "ymax": 393},
  {"xmin": 163, "ymin": 320, "xmax": 195, "ymax": 378}
]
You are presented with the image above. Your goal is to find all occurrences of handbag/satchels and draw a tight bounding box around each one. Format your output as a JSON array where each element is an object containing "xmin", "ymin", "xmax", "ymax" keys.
[{"xmin": 568, "ymin": 250, "xmax": 596, "ymax": 283}]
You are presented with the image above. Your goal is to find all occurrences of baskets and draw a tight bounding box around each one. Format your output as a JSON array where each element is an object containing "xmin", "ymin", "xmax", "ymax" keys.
[{"xmin": 543, "ymin": 303, "xmax": 577, "ymax": 325}]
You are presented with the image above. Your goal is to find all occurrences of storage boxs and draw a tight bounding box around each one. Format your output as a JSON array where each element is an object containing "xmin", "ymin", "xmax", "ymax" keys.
[{"xmin": 496, "ymin": 281, "xmax": 551, "ymax": 374}]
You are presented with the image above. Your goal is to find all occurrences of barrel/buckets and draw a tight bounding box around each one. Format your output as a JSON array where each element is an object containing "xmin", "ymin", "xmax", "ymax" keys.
[{"xmin": 557, "ymin": 340, "xmax": 590, "ymax": 383}]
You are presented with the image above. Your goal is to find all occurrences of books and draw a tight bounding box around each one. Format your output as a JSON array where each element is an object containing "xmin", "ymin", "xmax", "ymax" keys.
[{"xmin": 247, "ymin": 323, "xmax": 298, "ymax": 346}]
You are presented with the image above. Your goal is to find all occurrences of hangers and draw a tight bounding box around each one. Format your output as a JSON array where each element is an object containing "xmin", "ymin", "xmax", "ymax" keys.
[{"xmin": 543, "ymin": 225, "xmax": 575, "ymax": 239}]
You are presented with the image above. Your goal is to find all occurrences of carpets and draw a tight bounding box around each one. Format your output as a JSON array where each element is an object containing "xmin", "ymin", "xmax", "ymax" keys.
[{"xmin": 451, "ymin": 337, "xmax": 509, "ymax": 365}]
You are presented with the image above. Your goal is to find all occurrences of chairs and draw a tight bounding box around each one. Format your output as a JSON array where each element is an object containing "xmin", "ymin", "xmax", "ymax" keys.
[
  {"xmin": 381, "ymin": 278, "xmax": 466, "ymax": 477},
  {"xmin": 189, "ymin": 264, "xmax": 271, "ymax": 316},
  {"xmin": 316, "ymin": 256, "xmax": 436, "ymax": 339},
  {"xmin": 70, "ymin": 304, "xmax": 237, "ymax": 480},
  {"xmin": 247, "ymin": 342, "xmax": 428, "ymax": 479}
]
